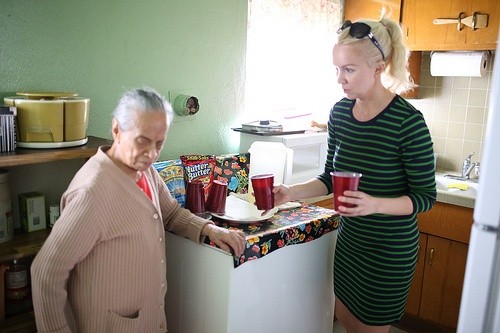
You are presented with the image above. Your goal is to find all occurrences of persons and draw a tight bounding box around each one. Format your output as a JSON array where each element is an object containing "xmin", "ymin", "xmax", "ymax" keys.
[
  {"xmin": 30, "ymin": 89, "xmax": 245, "ymax": 333},
  {"xmin": 251, "ymin": 19, "xmax": 437, "ymax": 333}
]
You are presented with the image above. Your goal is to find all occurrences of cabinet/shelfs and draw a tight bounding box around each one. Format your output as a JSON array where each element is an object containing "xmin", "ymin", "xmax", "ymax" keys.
[
  {"xmin": 340, "ymin": 1, "xmax": 500, "ymax": 51},
  {"xmin": 404, "ymin": 202, "xmax": 474, "ymax": 331},
  {"xmin": 0, "ymin": 136, "xmax": 119, "ymax": 321}
]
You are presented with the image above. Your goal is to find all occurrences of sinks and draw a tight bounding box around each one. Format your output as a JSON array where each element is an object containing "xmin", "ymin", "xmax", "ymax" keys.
[
  {"xmin": 449, "ymin": 182, "xmax": 479, "ymax": 208},
  {"xmin": 434, "ymin": 176, "xmax": 460, "ymax": 204}
]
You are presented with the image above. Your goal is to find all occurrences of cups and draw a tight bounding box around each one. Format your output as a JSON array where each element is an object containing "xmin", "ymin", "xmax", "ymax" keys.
[
  {"xmin": 250, "ymin": 174, "xmax": 274, "ymax": 210},
  {"xmin": 330, "ymin": 171, "xmax": 362, "ymax": 213},
  {"xmin": 205, "ymin": 180, "xmax": 228, "ymax": 215},
  {"xmin": 185, "ymin": 180, "xmax": 206, "ymax": 214}
]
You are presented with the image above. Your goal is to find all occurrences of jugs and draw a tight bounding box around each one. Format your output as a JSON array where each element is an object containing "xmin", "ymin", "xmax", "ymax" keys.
[{"xmin": 248, "ymin": 141, "xmax": 294, "ymax": 203}]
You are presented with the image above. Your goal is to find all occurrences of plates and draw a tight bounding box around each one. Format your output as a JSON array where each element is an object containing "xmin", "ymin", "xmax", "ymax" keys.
[
  {"xmin": 16, "ymin": 136, "xmax": 88, "ymax": 148},
  {"xmin": 195, "ymin": 213, "xmax": 212, "ymax": 220},
  {"xmin": 210, "ymin": 193, "xmax": 274, "ymax": 223}
]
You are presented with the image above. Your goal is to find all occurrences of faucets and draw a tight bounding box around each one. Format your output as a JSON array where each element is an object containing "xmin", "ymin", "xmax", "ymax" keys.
[{"xmin": 461, "ymin": 152, "xmax": 481, "ymax": 181}]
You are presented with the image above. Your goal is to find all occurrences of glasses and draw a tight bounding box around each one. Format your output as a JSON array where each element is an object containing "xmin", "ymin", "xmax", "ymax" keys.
[{"xmin": 341, "ymin": 20, "xmax": 386, "ymax": 62}]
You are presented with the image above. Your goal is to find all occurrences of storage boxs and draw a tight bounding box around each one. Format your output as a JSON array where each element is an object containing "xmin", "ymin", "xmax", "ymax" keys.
[
  {"xmin": 214, "ymin": 153, "xmax": 251, "ymax": 195},
  {"xmin": 19, "ymin": 192, "xmax": 47, "ymax": 235}
]
